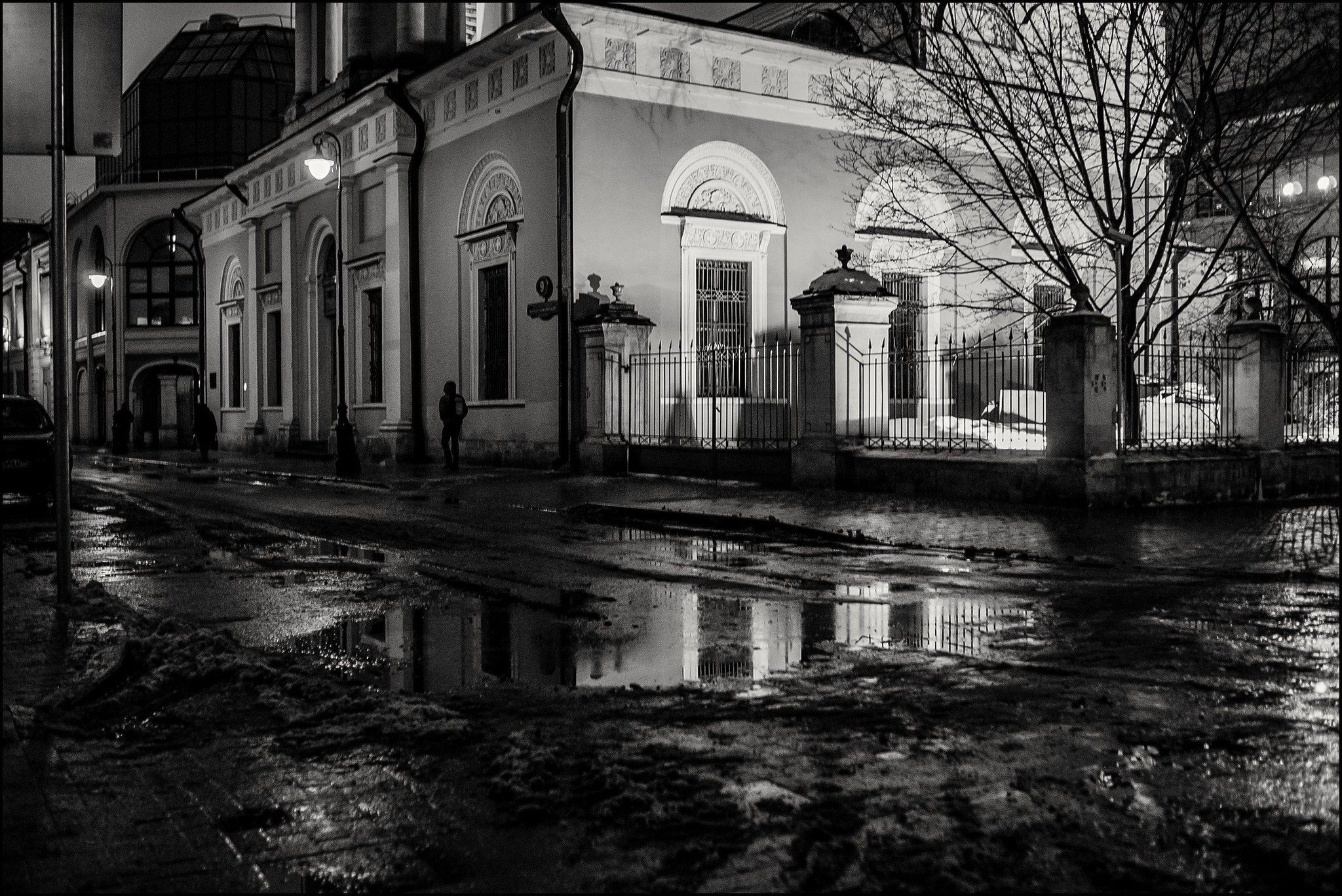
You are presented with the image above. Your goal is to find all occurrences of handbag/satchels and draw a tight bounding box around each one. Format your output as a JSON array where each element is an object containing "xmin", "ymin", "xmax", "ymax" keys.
[
  {"xmin": 455, "ymin": 396, "xmax": 463, "ymax": 415},
  {"xmin": 210, "ymin": 434, "xmax": 219, "ymax": 451}
]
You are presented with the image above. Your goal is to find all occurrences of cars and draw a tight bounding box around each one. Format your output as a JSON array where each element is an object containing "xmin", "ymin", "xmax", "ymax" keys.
[{"xmin": 0, "ymin": 393, "xmax": 73, "ymax": 503}]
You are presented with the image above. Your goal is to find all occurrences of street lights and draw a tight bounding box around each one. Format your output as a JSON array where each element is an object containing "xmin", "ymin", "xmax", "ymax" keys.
[
  {"xmin": 87, "ymin": 253, "xmax": 128, "ymax": 455},
  {"xmin": 2, "ymin": 315, "xmax": 10, "ymax": 396},
  {"xmin": 305, "ymin": 131, "xmax": 359, "ymax": 477}
]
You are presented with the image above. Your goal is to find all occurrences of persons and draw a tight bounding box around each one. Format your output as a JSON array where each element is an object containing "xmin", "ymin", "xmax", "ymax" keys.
[
  {"xmin": 113, "ymin": 402, "xmax": 134, "ymax": 454},
  {"xmin": 192, "ymin": 398, "xmax": 218, "ymax": 462},
  {"xmin": 439, "ymin": 380, "xmax": 468, "ymax": 471}
]
